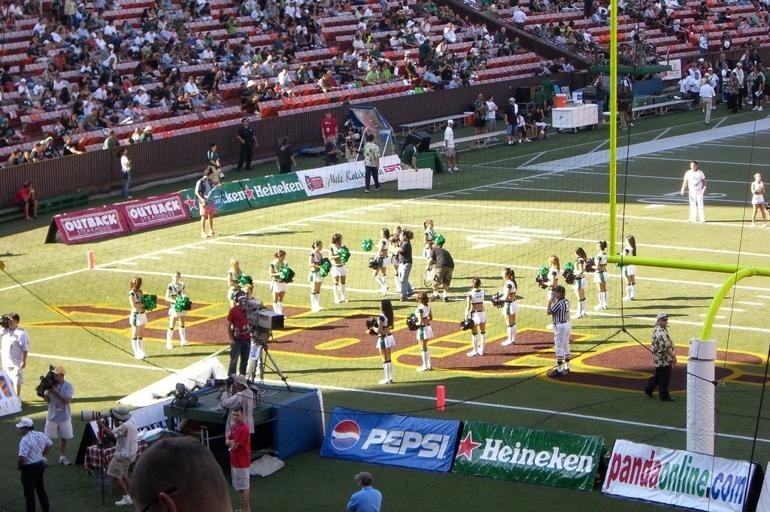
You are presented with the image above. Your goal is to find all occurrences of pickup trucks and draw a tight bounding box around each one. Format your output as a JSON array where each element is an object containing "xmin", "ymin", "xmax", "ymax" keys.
[{"xmin": 573, "ymin": 74, "xmax": 663, "ymax": 113}]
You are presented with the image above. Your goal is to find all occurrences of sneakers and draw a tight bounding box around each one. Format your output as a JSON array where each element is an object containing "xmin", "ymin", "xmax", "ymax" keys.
[
  {"xmin": 644, "ymin": 389, "xmax": 676, "ymax": 401},
  {"xmin": 309, "ymin": 277, "xmax": 637, "ymax": 385},
  {"xmin": 166, "ymin": 340, "xmax": 189, "ymax": 351},
  {"xmin": 689, "ymin": 218, "xmax": 705, "ymax": 224},
  {"xmin": 59, "ymin": 456, "xmax": 71, "ymax": 466},
  {"xmin": 683, "ymin": 98, "xmax": 764, "ymax": 126},
  {"xmin": 114, "ymin": 495, "xmax": 133, "ymax": 507},
  {"xmin": 364, "ymin": 187, "xmax": 381, "ymax": 193},
  {"xmin": 448, "ymin": 167, "xmax": 462, "ymax": 175},
  {"xmin": 750, "ymin": 222, "xmax": 768, "ymax": 229},
  {"xmin": 135, "ymin": 350, "xmax": 146, "ymax": 360},
  {"xmin": 201, "ymin": 230, "xmax": 215, "ymax": 239},
  {"xmin": 619, "ymin": 122, "xmax": 635, "ymax": 132},
  {"xmin": 475, "ymin": 134, "xmax": 550, "ymax": 146}
]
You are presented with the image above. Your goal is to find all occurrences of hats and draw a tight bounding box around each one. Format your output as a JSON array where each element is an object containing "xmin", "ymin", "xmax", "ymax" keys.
[
  {"xmin": 551, "ymin": 285, "xmax": 565, "ymax": 293},
  {"xmin": 354, "ymin": 472, "xmax": 372, "ymax": 485},
  {"xmin": 656, "ymin": 312, "xmax": 667, "ymax": 321},
  {"xmin": 15, "ymin": 418, "xmax": 34, "ymax": 429},
  {"xmin": 232, "ymin": 373, "xmax": 248, "ymax": 389},
  {"xmin": 112, "ymin": 407, "xmax": 130, "ymax": 421},
  {"xmin": 51, "ymin": 366, "xmax": 65, "ymax": 375}
]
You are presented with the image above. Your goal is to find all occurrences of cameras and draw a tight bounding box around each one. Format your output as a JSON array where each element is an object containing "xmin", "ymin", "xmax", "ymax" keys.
[{"xmin": 207, "ymin": 378, "xmax": 234, "ymax": 388}]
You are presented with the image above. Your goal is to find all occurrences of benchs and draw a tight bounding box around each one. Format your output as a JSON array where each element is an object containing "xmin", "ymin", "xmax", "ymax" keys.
[{"xmin": 603, "ymin": 100, "xmax": 694, "ymax": 122}]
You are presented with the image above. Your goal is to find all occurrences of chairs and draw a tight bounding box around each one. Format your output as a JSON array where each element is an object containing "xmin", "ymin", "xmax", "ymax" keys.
[
  {"xmin": 0, "ymin": 0, "xmax": 597, "ymax": 170},
  {"xmin": 476, "ymin": 1, "xmax": 769, "ymax": 62}
]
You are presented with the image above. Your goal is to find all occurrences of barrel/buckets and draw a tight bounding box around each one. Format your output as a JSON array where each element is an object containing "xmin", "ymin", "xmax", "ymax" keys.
[
  {"xmin": 571, "ymin": 91, "xmax": 583, "ymax": 104},
  {"xmin": 556, "ymin": 94, "xmax": 567, "ymax": 107}
]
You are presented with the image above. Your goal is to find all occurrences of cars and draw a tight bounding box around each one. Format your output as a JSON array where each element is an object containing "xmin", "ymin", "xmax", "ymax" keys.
[{"xmin": 719, "ymin": 57, "xmax": 770, "ymax": 96}]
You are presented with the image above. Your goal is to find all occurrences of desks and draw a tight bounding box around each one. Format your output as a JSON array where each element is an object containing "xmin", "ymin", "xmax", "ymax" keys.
[{"xmin": 84, "ymin": 443, "xmax": 147, "ymax": 476}]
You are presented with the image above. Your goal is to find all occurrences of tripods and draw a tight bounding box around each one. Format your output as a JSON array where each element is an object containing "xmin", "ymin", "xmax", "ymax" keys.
[{"xmin": 250, "ymin": 344, "xmax": 292, "ymax": 393}]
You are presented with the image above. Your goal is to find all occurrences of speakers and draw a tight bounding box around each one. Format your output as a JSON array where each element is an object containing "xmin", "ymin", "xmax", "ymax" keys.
[{"xmin": 517, "ymin": 87, "xmax": 531, "ymax": 103}]
